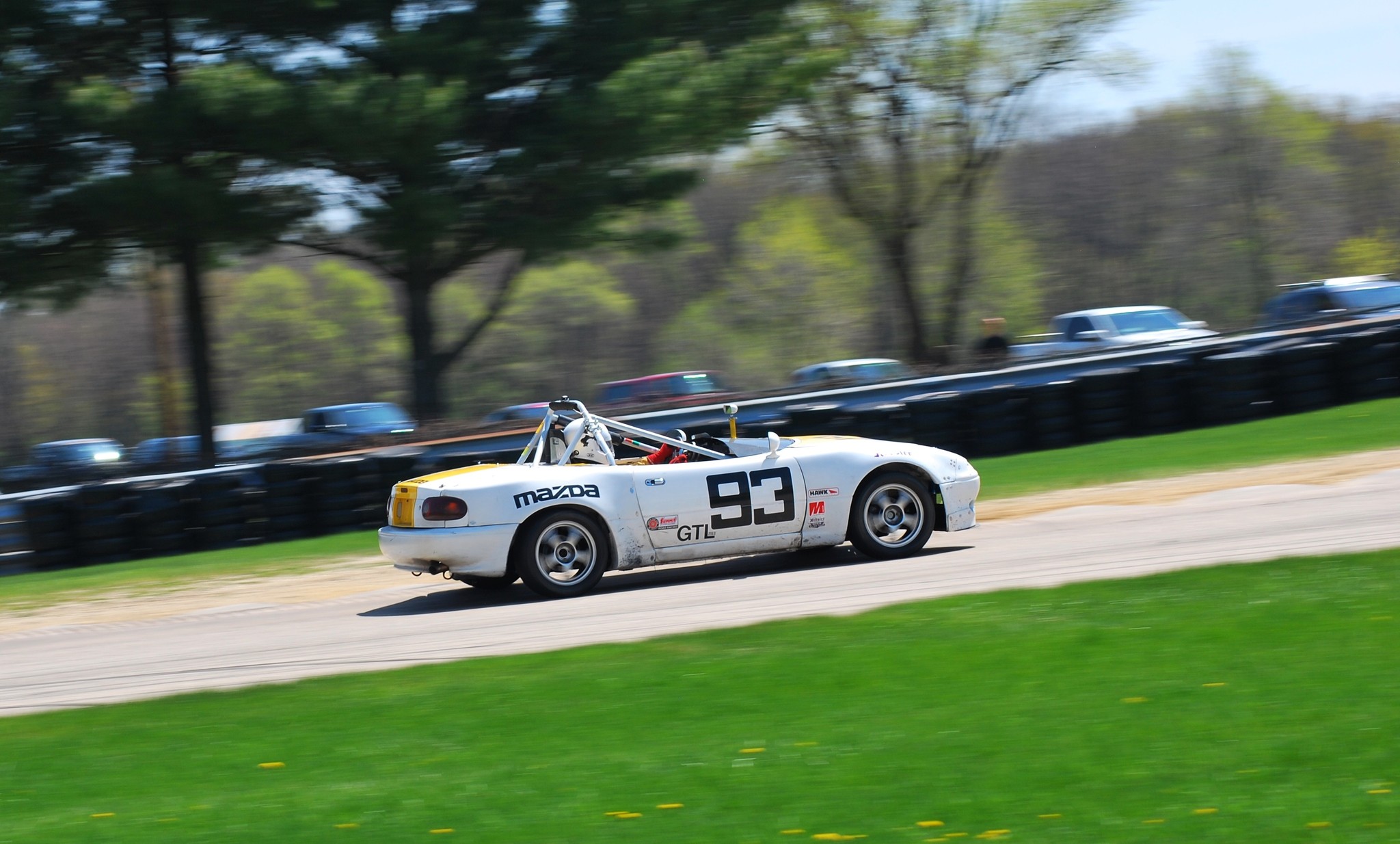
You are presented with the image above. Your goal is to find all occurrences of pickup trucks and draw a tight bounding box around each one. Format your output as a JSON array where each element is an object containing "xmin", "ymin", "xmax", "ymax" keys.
[{"xmin": 1005, "ymin": 305, "xmax": 1224, "ymax": 382}]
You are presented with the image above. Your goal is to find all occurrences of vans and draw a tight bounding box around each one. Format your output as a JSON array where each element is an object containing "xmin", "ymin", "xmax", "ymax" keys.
[
  {"xmin": 595, "ymin": 369, "xmax": 730, "ymax": 417},
  {"xmin": 1258, "ymin": 274, "xmax": 1400, "ymax": 330}
]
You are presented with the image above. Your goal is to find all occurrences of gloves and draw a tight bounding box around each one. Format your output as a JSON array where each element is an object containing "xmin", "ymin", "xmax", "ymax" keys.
[
  {"xmin": 669, "ymin": 454, "xmax": 688, "ymax": 464},
  {"xmin": 647, "ymin": 443, "xmax": 679, "ymax": 464}
]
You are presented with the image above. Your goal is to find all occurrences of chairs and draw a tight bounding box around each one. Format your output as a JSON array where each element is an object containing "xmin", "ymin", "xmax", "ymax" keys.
[{"xmin": 548, "ymin": 430, "xmax": 571, "ymax": 465}]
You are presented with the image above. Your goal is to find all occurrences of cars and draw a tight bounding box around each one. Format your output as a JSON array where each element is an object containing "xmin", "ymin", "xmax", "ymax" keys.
[
  {"xmin": 0, "ymin": 400, "xmax": 418, "ymax": 500},
  {"xmin": 378, "ymin": 396, "xmax": 981, "ymax": 601},
  {"xmin": 785, "ymin": 357, "xmax": 925, "ymax": 411},
  {"xmin": 480, "ymin": 401, "xmax": 577, "ymax": 423}
]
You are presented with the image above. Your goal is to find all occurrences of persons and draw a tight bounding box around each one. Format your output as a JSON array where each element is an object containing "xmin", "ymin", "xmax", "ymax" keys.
[
  {"xmin": 561, "ymin": 416, "xmax": 688, "ymax": 466},
  {"xmin": 972, "ymin": 316, "xmax": 1009, "ymax": 365}
]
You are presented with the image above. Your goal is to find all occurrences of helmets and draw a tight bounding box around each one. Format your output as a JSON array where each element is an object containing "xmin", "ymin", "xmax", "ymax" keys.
[{"xmin": 564, "ymin": 418, "xmax": 615, "ymax": 464}]
